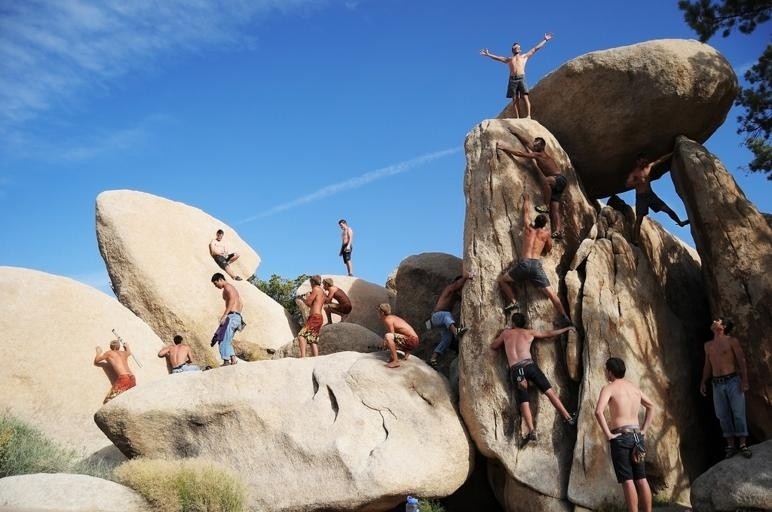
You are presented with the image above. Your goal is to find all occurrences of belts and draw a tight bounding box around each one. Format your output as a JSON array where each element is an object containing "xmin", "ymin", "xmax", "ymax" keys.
[
  {"xmin": 713, "ymin": 375, "xmax": 736, "ymax": 383},
  {"xmin": 173, "ymin": 363, "xmax": 185, "ymax": 368},
  {"xmin": 230, "ymin": 312, "xmax": 240, "ymax": 314},
  {"xmin": 611, "ymin": 429, "xmax": 639, "ymax": 434}
]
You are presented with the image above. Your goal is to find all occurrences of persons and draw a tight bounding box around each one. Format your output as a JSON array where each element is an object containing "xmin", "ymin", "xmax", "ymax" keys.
[
  {"xmin": 376, "ymin": 303, "xmax": 420, "ymax": 367},
  {"xmin": 322, "ymin": 278, "xmax": 352, "ymax": 326},
  {"xmin": 595, "ymin": 357, "xmax": 656, "ymax": 512},
  {"xmin": 94, "ymin": 340, "xmax": 137, "ymax": 405},
  {"xmin": 499, "ymin": 192, "xmax": 571, "ymax": 324},
  {"xmin": 480, "ymin": 31, "xmax": 555, "ymax": 119},
  {"xmin": 211, "ymin": 273, "xmax": 243, "ymax": 367},
  {"xmin": 297, "ymin": 275, "xmax": 325, "ymax": 357},
  {"xmin": 158, "ymin": 335, "xmax": 210, "ymax": 372},
  {"xmin": 625, "ymin": 149, "xmax": 690, "ymax": 245},
  {"xmin": 491, "ymin": 313, "xmax": 578, "ymax": 439},
  {"xmin": 430, "ymin": 271, "xmax": 473, "ymax": 372},
  {"xmin": 209, "ymin": 229, "xmax": 243, "ymax": 281},
  {"xmin": 339, "ymin": 220, "xmax": 354, "ymax": 276},
  {"xmin": 496, "ymin": 127, "xmax": 567, "ymax": 236},
  {"xmin": 700, "ymin": 317, "xmax": 753, "ymax": 458}
]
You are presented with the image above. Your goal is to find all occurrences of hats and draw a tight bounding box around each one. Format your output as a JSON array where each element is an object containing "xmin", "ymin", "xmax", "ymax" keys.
[
  {"xmin": 110, "ymin": 340, "xmax": 120, "ymax": 346},
  {"xmin": 312, "ymin": 276, "xmax": 321, "ymax": 284},
  {"xmin": 324, "ymin": 278, "xmax": 333, "ymax": 285},
  {"xmin": 380, "ymin": 304, "xmax": 391, "ymax": 314}
]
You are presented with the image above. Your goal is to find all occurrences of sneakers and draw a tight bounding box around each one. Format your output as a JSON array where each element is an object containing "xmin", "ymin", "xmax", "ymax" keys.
[
  {"xmin": 455, "ymin": 328, "xmax": 467, "ymax": 335},
  {"xmin": 536, "ymin": 204, "xmax": 547, "ymax": 212},
  {"xmin": 552, "ymin": 231, "xmax": 560, "ymax": 237},
  {"xmin": 740, "ymin": 446, "xmax": 752, "ymax": 457},
  {"xmin": 430, "ymin": 360, "xmax": 439, "ymax": 371},
  {"xmin": 680, "ymin": 221, "xmax": 689, "ymax": 226},
  {"xmin": 505, "ymin": 301, "xmax": 518, "ymax": 310},
  {"xmin": 526, "ymin": 432, "xmax": 536, "ymax": 439},
  {"xmin": 569, "ymin": 411, "xmax": 578, "ymax": 425},
  {"xmin": 725, "ymin": 448, "xmax": 736, "ymax": 458}
]
